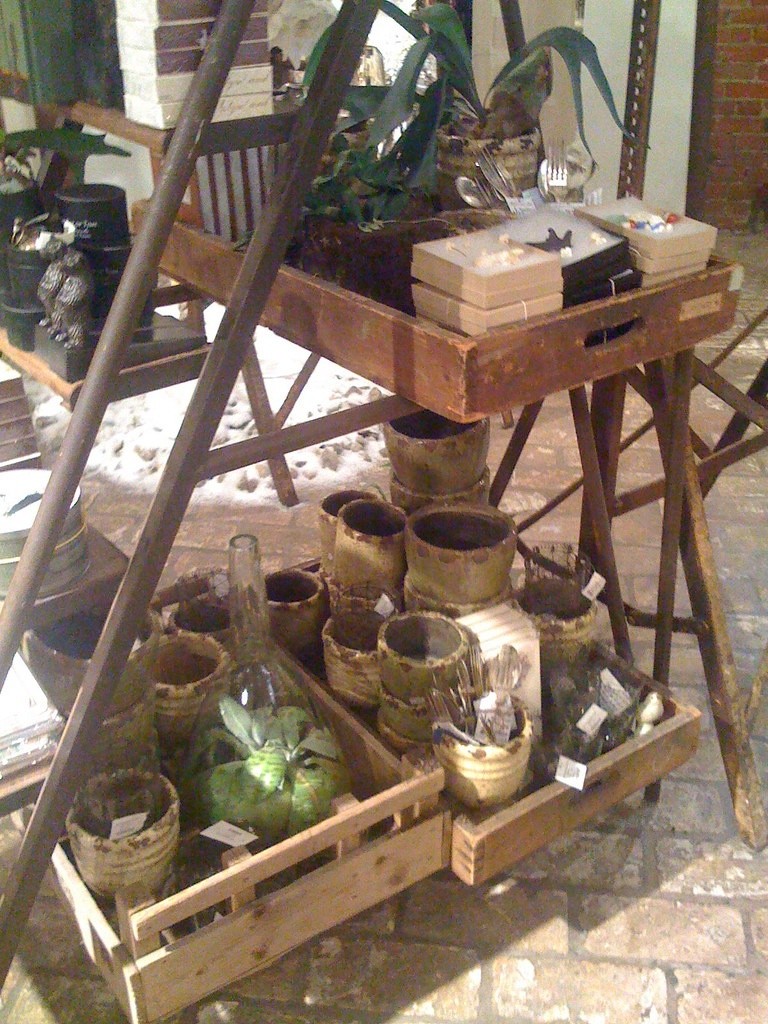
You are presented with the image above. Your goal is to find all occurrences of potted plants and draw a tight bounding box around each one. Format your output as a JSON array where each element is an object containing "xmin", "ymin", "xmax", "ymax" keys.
[{"xmin": 324, "ymin": 0, "xmax": 651, "ymax": 229}]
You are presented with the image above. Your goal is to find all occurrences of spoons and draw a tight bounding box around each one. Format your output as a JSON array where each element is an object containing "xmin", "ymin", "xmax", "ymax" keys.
[
  {"xmin": 538, "ymin": 157, "xmax": 555, "ymax": 202},
  {"xmin": 456, "ymin": 176, "xmax": 486, "ymax": 210}
]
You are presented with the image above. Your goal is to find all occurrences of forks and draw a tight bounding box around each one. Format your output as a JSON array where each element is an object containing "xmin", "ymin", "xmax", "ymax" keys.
[
  {"xmin": 548, "ymin": 136, "xmax": 569, "ymax": 202},
  {"xmin": 426, "ymin": 642, "xmax": 530, "ymax": 743},
  {"xmin": 472, "ymin": 147, "xmax": 518, "ymax": 212}
]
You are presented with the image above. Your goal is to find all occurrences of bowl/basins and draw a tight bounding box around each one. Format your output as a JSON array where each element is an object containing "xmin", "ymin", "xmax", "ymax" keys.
[
  {"xmin": 20, "ymin": 601, "xmax": 241, "ymax": 906},
  {"xmin": 269, "ymin": 408, "xmax": 600, "ymax": 810}
]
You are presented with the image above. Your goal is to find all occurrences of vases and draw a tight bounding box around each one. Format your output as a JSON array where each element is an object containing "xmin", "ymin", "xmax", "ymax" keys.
[{"xmin": 13, "ymin": 406, "xmax": 606, "ymax": 905}]
